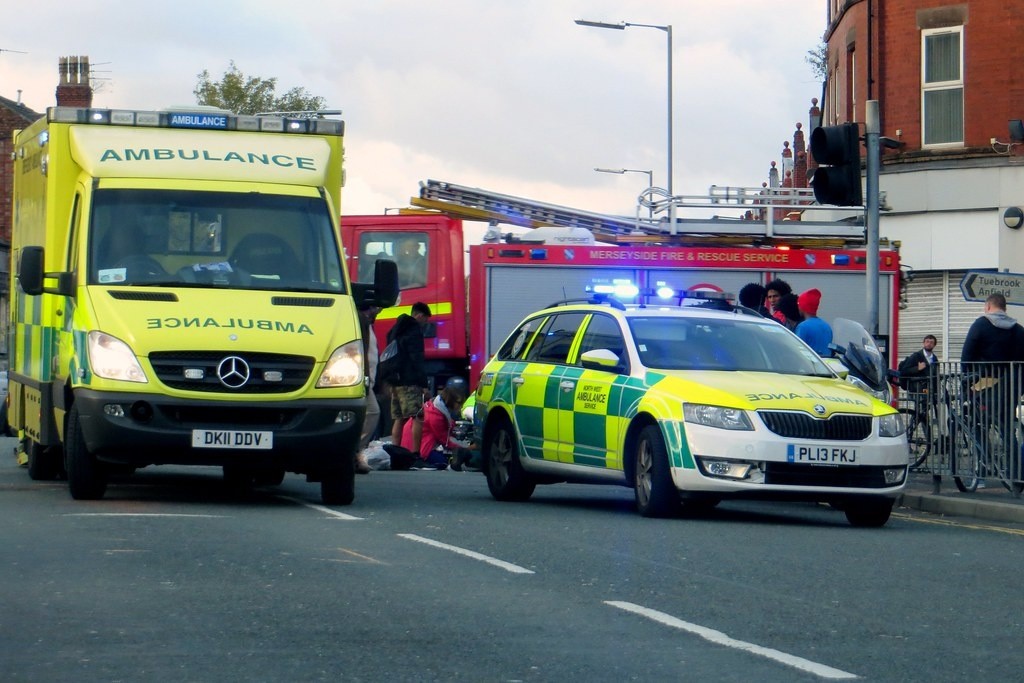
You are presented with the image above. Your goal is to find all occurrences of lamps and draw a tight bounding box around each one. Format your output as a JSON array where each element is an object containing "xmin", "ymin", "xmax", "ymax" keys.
[
  {"xmin": 879, "ymin": 136, "xmax": 906, "ymax": 150},
  {"xmin": 1006, "ymin": 119, "xmax": 1024, "ymax": 144},
  {"xmin": 1003, "ymin": 206, "xmax": 1024, "ymax": 229}
]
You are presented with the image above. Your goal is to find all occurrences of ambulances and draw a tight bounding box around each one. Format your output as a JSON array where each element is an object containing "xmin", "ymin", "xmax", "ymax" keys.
[
  {"xmin": 7, "ymin": 105, "xmax": 399, "ymax": 504},
  {"xmin": 474, "ymin": 294, "xmax": 909, "ymax": 528}
]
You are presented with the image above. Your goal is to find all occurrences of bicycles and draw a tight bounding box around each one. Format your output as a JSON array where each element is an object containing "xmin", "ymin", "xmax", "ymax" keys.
[{"xmin": 898, "ymin": 374, "xmax": 1024, "ymax": 495}]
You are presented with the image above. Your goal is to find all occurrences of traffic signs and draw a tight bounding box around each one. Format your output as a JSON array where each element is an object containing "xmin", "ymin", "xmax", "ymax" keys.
[{"xmin": 959, "ymin": 271, "xmax": 1024, "ymax": 307}]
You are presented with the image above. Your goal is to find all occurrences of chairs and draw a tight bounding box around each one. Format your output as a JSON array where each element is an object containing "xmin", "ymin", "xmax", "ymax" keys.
[
  {"xmin": 96, "ymin": 224, "xmax": 150, "ymax": 274},
  {"xmin": 229, "ymin": 231, "xmax": 302, "ymax": 279}
]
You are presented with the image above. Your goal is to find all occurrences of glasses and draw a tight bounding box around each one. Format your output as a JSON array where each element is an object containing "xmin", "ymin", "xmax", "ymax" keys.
[{"xmin": 456, "ymin": 400, "xmax": 461, "ymax": 406}]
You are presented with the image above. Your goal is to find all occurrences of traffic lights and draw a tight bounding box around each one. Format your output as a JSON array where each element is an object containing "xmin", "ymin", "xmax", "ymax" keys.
[{"xmin": 809, "ymin": 121, "xmax": 864, "ymax": 208}]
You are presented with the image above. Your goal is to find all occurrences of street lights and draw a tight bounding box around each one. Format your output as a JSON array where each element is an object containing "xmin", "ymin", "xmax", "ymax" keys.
[
  {"xmin": 595, "ymin": 167, "xmax": 652, "ymax": 222},
  {"xmin": 576, "ymin": 17, "xmax": 676, "ymax": 218}
]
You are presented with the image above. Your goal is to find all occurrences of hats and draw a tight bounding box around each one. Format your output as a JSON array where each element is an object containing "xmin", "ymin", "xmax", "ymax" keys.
[
  {"xmin": 383, "ymin": 444, "xmax": 415, "ymax": 471},
  {"xmin": 797, "ymin": 289, "xmax": 821, "ymax": 315}
]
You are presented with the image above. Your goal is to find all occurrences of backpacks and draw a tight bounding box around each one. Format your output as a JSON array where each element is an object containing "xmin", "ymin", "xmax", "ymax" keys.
[{"xmin": 898, "ymin": 352, "xmax": 923, "ymax": 390}]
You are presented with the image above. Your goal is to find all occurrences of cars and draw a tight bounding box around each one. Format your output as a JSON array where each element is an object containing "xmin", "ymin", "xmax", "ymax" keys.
[{"xmin": 0, "ymin": 371, "xmax": 19, "ymax": 437}]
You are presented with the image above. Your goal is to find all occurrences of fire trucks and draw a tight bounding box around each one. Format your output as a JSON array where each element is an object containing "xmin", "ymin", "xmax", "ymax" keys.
[{"xmin": 339, "ymin": 180, "xmax": 914, "ymax": 436}]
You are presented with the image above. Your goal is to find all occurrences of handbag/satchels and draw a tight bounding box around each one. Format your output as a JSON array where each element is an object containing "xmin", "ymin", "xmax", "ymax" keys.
[{"xmin": 379, "ymin": 340, "xmax": 401, "ymax": 378}]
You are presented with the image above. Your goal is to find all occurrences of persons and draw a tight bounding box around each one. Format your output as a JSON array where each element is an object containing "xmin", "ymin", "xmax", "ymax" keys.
[
  {"xmin": 901, "ymin": 334, "xmax": 941, "ymax": 454},
  {"xmin": 353, "ymin": 324, "xmax": 380, "ymax": 475},
  {"xmin": 398, "ymin": 385, "xmax": 478, "ymax": 469},
  {"xmin": 960, "ymin": 293, "xmax": 1024, "ymax": 489},
  {"xmin": 378, "ymin": 301, "xmax": 432, "ymax": 470},
  {"xmin": 739, "ymin": 277, "xmax": 834, "ymax": 358}
]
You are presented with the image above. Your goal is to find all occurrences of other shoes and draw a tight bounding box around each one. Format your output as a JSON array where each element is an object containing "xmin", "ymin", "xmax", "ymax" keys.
[{"xmin": 356, "ymin": 452, "xmax": 371, "ymax": 474}]
